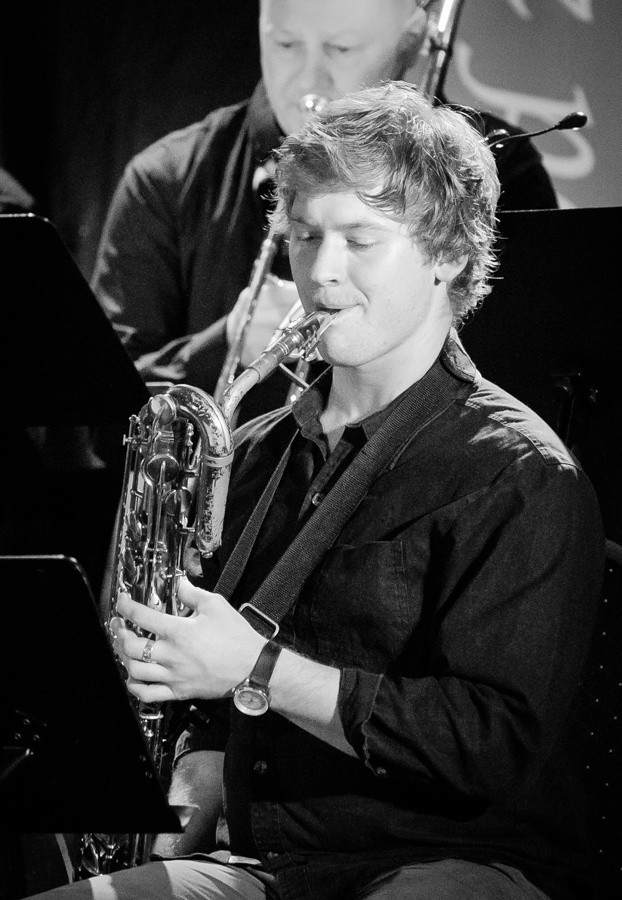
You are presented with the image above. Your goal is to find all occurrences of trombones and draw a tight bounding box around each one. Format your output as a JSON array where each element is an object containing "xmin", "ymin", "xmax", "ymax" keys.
[{"xmin": 211, "ymin": 0, "xmax": 461, "ymax": 408}]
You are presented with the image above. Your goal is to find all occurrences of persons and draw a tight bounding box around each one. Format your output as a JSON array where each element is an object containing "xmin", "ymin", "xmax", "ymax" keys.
[
  {"xmin": 19, "ymin": 81, "xmax": 607, "ymax": 900},
  {"xmin": 80, "ymin": 0, "xmax": 560, "ymax": 431}
]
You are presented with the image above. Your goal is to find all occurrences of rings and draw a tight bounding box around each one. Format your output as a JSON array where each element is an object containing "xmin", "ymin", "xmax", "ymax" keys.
[{"xmin": 141, "ymin": 638, "xmax": 155, "ymax": 662}]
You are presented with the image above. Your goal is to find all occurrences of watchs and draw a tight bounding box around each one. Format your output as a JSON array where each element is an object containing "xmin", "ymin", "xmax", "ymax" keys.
[{"xmin": 230, "ymin": 639, "xmax": 283, "ymax": 719}]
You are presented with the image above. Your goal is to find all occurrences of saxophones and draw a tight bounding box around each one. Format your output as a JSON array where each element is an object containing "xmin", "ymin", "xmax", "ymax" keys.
[{"xmin": 72, "ymin": 308, "xmax": 340, "ymax": 886}]
